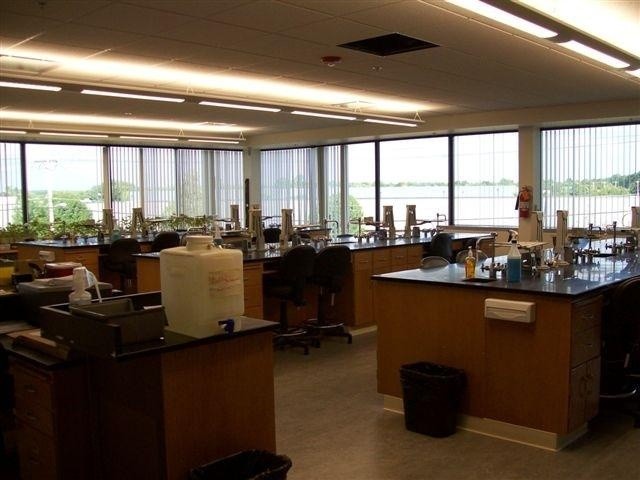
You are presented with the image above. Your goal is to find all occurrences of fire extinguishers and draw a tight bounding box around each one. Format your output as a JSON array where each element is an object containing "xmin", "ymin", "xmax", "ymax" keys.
[{"xmin": 515, "ymin": 185, "xmax": 531, "ymax": 217}]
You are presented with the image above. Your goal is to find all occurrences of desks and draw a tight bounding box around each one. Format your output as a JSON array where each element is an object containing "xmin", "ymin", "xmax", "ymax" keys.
[
  {"xmin": 0, "ymin": 297, "xmax": 279, "ymax": 478},
  {"xmin": 11, "ymin": 232, "xmax": 243, "ymax": 282},
  {"xmin": 370, "ymin": 237, "xmax": 638, "ymax": 452},
  {"xmin": 133, "ymin": 233, "xmax": 497, "ymax": 330}
]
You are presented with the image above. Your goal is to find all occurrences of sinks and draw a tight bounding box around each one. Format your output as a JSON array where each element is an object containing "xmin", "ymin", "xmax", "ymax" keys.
[
  {"xmin": 463, "ymin": 276, "xmax": 497, "ymax": 284},
  {"xmin": 593, "ymin": 251, "xmax": 616, "ymax": 259},
  {"xmin": 332, "ymin": 240, "xmax": 355, "ymax": 245},
  {"xmin": 336, "ymin": 234, "xmax": 353, "ymax": 238}
]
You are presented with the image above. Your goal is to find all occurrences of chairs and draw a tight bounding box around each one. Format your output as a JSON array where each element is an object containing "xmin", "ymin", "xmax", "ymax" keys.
[
  {"xmin": 100, "ymin": 232, "xmax": 180, "ymax": 295},
  {"xmin": 598, "ymin": 276, "xmax": 640, "ymax": 429},
  {"xmin": 269, "ymin": 245, "xmax": 353, "ymax": 355},
  {"xmin": 263, "ymin": 227, "xmax": 282, "ymax": 243}
]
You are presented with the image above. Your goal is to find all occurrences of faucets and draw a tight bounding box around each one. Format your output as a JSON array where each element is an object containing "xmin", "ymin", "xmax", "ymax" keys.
[
  {"xmin": 435, "ymin": 214, "xmax": 446, "ymax": 236},
  {"xmin": 606, "ymin": 220, "xmax": 617, "ymax": 253},
  {"xmin": 324, "ymin": 219, "xmax": 339, "ymax": 240},
  {"xmin": 476, "ymin": 231, "xmax": 498, "ymax": 278},
  {"xmin": 261, "ymin": 216, "xmax": 273, "ymax": 220},
  {"xmin": 585, "ymin": 223, "xmax": 602, "ymax": 256},
  {"xmin": 217, "ymin": 319, "xmax": 236, "ymax": 335},
  {"xmin": 350, "ymin": 217, "xmax": 361, "ymax": 237}
]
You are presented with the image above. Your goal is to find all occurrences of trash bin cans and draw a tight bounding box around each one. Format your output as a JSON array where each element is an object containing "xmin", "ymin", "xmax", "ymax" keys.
[
  {"xmin": 188, "ymin": 448, "xmax": 292, "ymax": 480},
  {"xmin": 400, "ymin": 362, "xmax": 465, "ymax": 438}
]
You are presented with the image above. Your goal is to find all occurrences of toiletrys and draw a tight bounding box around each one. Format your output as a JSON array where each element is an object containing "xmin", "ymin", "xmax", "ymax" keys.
[{"xmin": 465, "ymin": 246, "xmax": 476, "ymax": 280}]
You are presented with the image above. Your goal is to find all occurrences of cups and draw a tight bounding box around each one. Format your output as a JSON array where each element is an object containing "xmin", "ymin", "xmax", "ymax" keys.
[
  {"xmin": 545, "ymin": 271, "xmax": 555, "ymax": 283},
  {"xmin": 546, "ymin": 248, "xmax": 554, "ymax": 265}
]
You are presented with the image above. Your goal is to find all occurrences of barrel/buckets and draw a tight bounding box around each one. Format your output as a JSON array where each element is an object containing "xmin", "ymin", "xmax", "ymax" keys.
[{"xmin": 160, "ymin": 238, "xmax": 245, "ymax": 342}]
